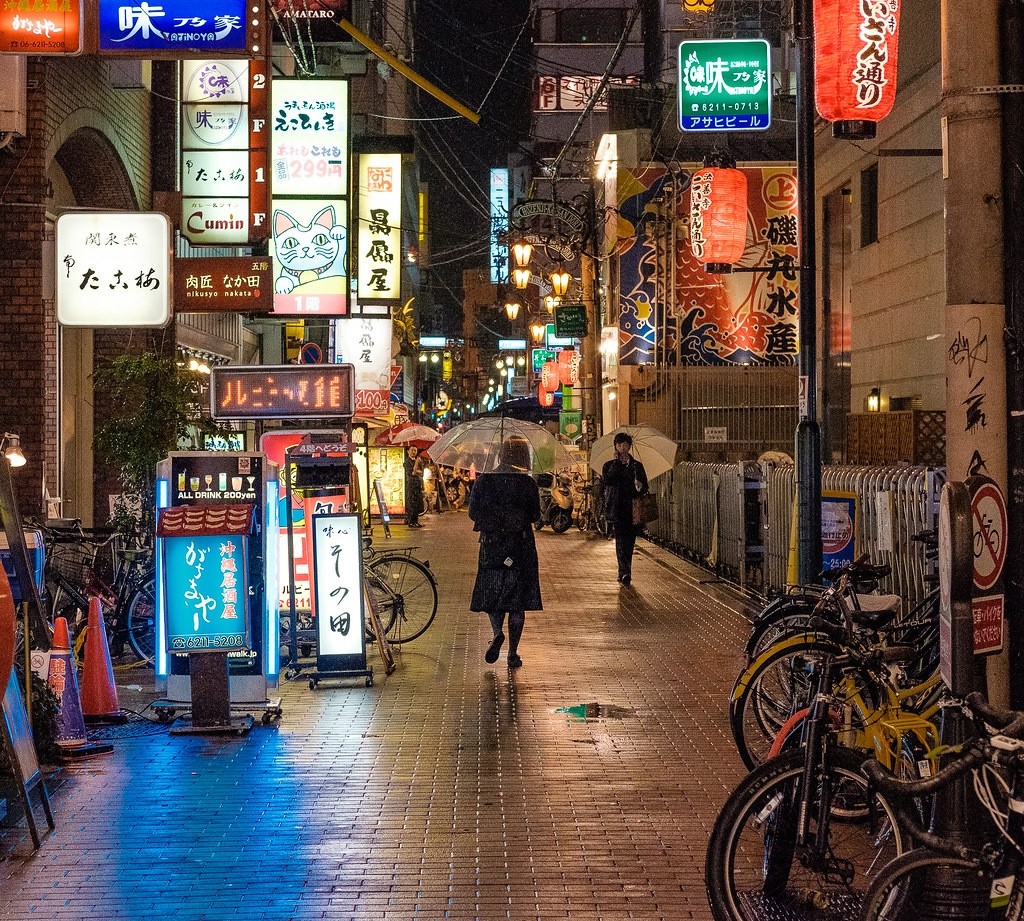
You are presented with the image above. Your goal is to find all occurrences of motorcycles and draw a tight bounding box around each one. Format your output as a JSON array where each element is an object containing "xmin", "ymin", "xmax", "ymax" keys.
[{"xmin": 532, "ymin": 471, "xmax": 574, "ymax": 533}]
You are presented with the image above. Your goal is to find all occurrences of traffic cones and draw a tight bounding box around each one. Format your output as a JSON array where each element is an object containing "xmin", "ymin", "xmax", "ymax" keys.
[
  {"xmin": 41, "ymin": 616, "xmax": 115, "ymax": 763},
  {"xmin": 81, "ymin": 596, "xmax": 129, "ymax": 727}
]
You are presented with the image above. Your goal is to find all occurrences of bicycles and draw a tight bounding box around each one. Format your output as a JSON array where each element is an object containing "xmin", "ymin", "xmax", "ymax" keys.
[
  {"xmin": 13, "ymin": 514, "xmax": 158, "ymax": 670},
  {"xmin": 704, "ymin": 526, "xmax": 1024, "ymax": 920},
  {"xmin": 362, "ymin": 537, "xmax": 439, "ymax": 655},
  {"xmin": 573, "ymin": 472, "xmax": 607, "ymax": 537}
]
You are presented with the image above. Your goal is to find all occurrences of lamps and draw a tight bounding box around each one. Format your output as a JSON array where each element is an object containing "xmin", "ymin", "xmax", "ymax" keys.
[
  {"xmin": 417, "ymin": 350, "xmax": 443, "ymax": 362},
  {"xmin": 0, "ymin": 432, "xmax": 28, "ymax": 468},
  {"xmin": 505, "ymin": 294, "xmax": 542, "ymax": 320},
  {"xmin": 511, "ymin": 267, "xmax": 553, "ymax": 289},
  {"xmin": 511, "ymin": 229, "xmax": 580, "ymax": 266},
  {"xmin": 530, "ymin": 319, "xmax": 555, "ymax": 341},
  {"xmin": 544, "ymin": 292, "xmax": 582, "ymax": 314},
  {"xmin": 544, "ymin": 233, "xmax": 592, "ymax": 297},
  {"xmin": 867, "ymin": 388, "xmax": 879, "ymax": 412}
]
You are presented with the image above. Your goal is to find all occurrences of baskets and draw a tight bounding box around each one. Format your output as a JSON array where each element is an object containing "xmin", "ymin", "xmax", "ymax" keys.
[{"xmin": 49, "ymin": 543, "xmax": 110, "ymax": 587}]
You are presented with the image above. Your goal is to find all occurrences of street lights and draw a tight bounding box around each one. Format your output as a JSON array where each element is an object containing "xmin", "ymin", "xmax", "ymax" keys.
[{"xmin": 480, "ymin": 184, "xmax": 605, "ymax": 447}]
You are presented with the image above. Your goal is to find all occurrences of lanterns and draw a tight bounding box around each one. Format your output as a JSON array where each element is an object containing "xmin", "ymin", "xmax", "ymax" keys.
[
  {"xmin": 814, "ymin": 0, "xmax": 899, "ymax": 140},
  {"xmin": 539, "ymin": 345, "xmax": 579, "ymax": 409},
  {"xmin": 691, "ymin": 154, "xmax": 747, "ymax": 275}
]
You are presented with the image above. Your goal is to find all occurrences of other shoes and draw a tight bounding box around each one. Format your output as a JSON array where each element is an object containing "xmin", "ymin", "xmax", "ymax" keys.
[
  {"xmin": 485, "ymin": 633, "xmax": 506, "ymax": 664},
  {"xmin": 409, "ymin": 522, "xmax": 421, "ymax": 528},
  {"xmin": 507, "ymin": 654, "xmax": 522, "ymax": 667},
  {"xmin": 622, "ymin": 574, "xmax": 631, "ymax": 585}
]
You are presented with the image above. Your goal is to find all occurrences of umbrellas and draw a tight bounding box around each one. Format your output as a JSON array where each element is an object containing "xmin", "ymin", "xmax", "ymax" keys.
[
  {"xmin": 374, "ymin": 418, "xmax": 494, "ymax": 463},
  {"xmin": 427, "ymin": 412, "xmax": 580, "ymax": 475},
  {"xmin": 590, "ymin": 424, "xmax": 678, "ymax": 484}
]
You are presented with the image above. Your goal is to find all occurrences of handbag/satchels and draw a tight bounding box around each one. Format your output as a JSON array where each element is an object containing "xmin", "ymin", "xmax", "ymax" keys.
[{"xmin": 632, "ymin": 491, "xmax": 659, "ymax": 525}]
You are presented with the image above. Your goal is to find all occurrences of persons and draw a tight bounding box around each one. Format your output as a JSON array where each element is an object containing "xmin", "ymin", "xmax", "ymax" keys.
[
  {"xmin": 404, "ymin": 445, "xmax": 425, "ymax": 528},
  {"xmin": 468, "ymin": 436, "xmax": 544, "ymax": 667},
  {"xmin": 601, "ymin": 433, "xmax": 649, "ymax": 580},
  {"xmin": 423, "ymin": 436, "xmax": 501, "ymax": 511}
]
